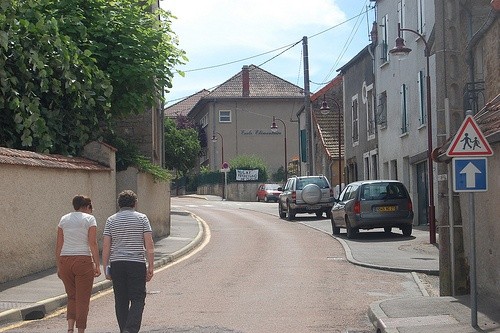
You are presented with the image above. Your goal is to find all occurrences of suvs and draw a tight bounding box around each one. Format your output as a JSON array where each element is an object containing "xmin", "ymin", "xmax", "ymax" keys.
[{"xmin": 277, "ymin": 174, "xmax": 334, "ymax": 221}]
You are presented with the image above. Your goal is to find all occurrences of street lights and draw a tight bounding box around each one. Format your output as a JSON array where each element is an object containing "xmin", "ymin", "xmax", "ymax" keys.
[
  {"xmin": 271, "ymin": 114, "xmax": 287, "ymax": 183},
  {"xmin": 319, "ymin": 94, "xmax": 342, "ymax": 194},
  {"xmin": 389, "ymin": 22, "xmax": 436, "ymax": 244},
  {"xmin": 211, "ymin": 128, "xmax": 225, "ymax": 199}
]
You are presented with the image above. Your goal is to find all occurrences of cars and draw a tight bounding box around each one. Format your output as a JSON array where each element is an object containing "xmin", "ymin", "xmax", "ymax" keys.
[
  {"xmin": 329, "ymin": 179, "xmax": 414, "ymax": 239},
  {"xmin": 255, "ymin": 183, "xmax": 284, "ymax": 203}
]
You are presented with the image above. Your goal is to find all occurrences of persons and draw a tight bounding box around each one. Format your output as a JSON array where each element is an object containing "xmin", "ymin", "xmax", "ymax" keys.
[
  {"xmin": 102, "ymin": 190, "xmax": 155, "ymax": 333},
  {"xmin": 55, "ymin": 195, "xmax": 101, "ymax": 333}
]
